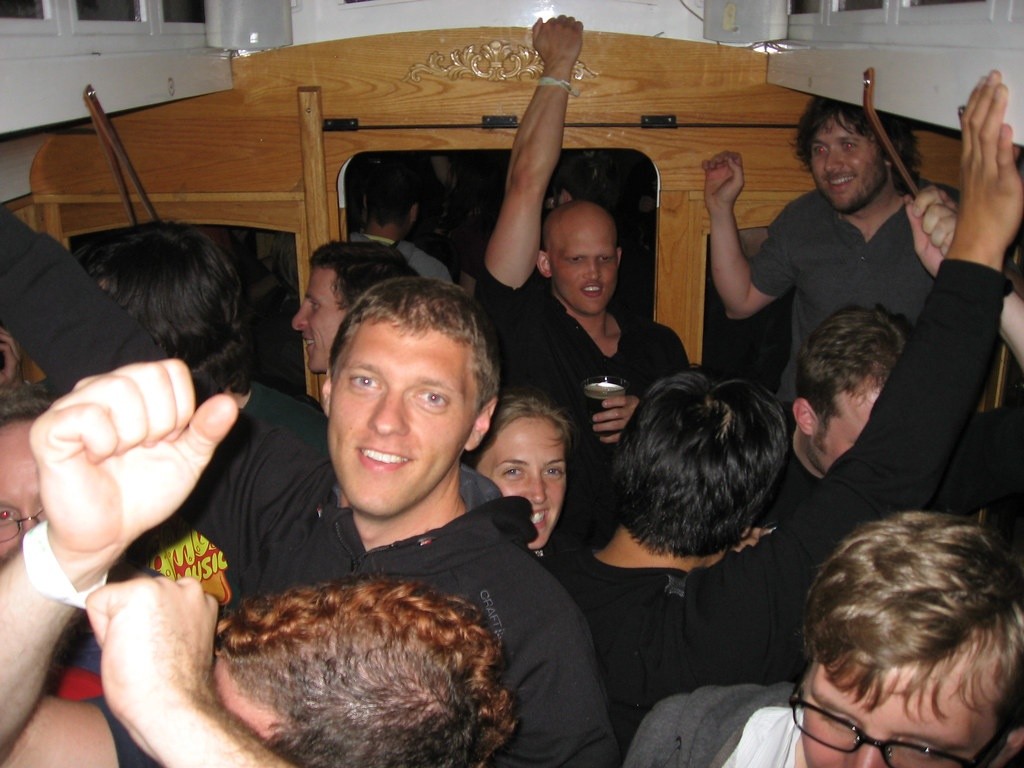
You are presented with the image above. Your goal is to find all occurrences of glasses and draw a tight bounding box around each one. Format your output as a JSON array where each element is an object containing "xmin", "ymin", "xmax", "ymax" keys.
[
  {"xmin": 788, "ymin": 657, "xmax": 1006, "ymax": 766},
  {"xmin": 0, "ymin": 501, "xmax": 47, "ymax": 543}
]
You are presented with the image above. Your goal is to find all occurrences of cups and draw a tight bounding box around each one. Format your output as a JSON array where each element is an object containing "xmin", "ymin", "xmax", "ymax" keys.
[{"xmin": 581, "ymin": 375, "xmax": 630, "ymax": 437}]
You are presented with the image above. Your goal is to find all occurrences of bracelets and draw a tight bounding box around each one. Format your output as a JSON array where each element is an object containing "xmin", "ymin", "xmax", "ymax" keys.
[
  {"xmin": 22, "ymin": 520, "xmax": 107, "ymax": 609},
  {"xmin": 536, "ymin": 76, "xmax": 580, "ymax": 97}
]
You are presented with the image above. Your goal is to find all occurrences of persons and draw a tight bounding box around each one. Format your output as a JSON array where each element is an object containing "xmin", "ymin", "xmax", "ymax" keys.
[{"xmin": 1, "ymin": 16, "xmax": 1024, "ymax": 768}]
